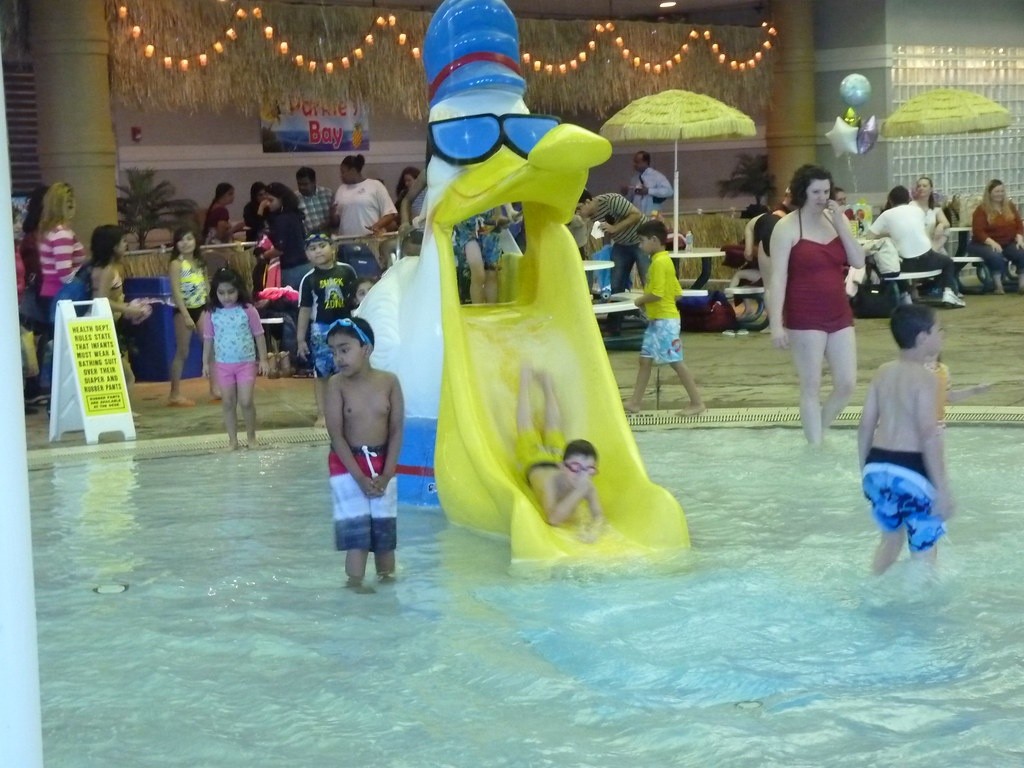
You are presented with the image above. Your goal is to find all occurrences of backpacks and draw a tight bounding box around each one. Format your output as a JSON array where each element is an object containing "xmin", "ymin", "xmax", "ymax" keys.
[{"xmin": 48, "ymin": 264, "xmax": 93, "ymax": 325}]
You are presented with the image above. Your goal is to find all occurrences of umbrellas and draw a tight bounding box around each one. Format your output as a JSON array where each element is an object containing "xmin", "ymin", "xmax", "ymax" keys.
[
  {"xmin": 882, "ymin": 88, "xmax": 1012, "ymax": 193},
  {"xmin": 597, "ymin": 89, "xmax": 758, "ymax": 252}
]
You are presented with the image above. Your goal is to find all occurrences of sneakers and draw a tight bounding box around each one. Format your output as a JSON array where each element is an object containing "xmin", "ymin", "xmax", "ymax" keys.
[
  {"xmin": 900, "ymin": 292, "xmax": 913, "ymax": 306},
  {"xmin": 942, "ymin": 291, "xmax": 966, "ymax": 308}
]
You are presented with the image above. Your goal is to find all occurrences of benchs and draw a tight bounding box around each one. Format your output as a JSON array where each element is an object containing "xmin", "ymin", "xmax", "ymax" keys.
[{"xmin": 590, "ymin": 254, "xmax": 1013, "ymax": 333}]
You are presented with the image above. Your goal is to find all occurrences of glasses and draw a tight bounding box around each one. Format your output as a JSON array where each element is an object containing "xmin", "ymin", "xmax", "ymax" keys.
[
  {"xmin": 577, "ymin": 205, "xmax": 583, "ymax": 210},
  {"xmin": 565, "ymin": 461, "xmax": 598, "ymax": 476},
  {"xmin": 309, "ymin": 233, "xmax": 331, "ymax": 240}
]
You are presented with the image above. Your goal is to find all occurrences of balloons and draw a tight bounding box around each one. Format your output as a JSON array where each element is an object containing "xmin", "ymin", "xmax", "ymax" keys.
[
  {"xmin": 858, "ymin": 114, "xmax": 879, "ymax": 155},
  {"xmin": 840, "ymin": 74, "xmax": 872, "ymax": 106},
  {"xmin": 842, "ymin": 106, "xmax": 863, "ymax": 127},
  {"xmin": 825, "ymin": 116, "xmax": 860, "ymax": 159}
]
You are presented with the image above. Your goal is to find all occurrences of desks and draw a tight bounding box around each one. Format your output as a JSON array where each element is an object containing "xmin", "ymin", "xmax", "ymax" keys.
[
  {"xmin": 948, "ymin": 226, "xmax": 974, "ymax": 257},
  {"xmin": 662, "ymin": 246, "xmax": 728, "ymax": 289},
  {"xmin": 582, "ymin": 259, "xmax": 616, "ymax": 292}
]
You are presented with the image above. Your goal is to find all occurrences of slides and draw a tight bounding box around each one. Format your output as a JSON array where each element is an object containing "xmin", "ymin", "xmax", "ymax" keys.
[{"xmin": 434, "ymin": 223, "xmax": 691, "ymax": 564}]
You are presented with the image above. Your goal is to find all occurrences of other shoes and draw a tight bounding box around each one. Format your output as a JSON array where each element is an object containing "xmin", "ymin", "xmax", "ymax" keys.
[
  {"xmin": 25, "ymin": 387, "xmax": 46, "ymax": 404},
  {"xmin": 995, "ymin": 289, "xmax": 1005, "ymax": 295}
]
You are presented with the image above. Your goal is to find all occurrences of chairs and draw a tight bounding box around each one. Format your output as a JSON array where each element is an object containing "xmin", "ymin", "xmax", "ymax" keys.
[
  {"xmin": 337, "ymin": 243, "xmax": 384, "ymax": 278},
  {"xmin": 123, "ymin": 273, "xmax": 204, "ymax": 385}
]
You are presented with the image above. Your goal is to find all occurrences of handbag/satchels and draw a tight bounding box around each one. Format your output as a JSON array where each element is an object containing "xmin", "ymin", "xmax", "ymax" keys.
[
  {"xmin": 854, "ymin": 264, "xmax": 901, "ymax": 318},
  {"xmin": 653, "ymin": 196, "xmax": 667, "ymax": 204},
  {"xmin": 680, "ymin": 300, "xmax": 738, "ymax": 333},
  {"xmin": 860, "ymin": 237, "xmax": 900, "ymax": 277},
  {"xmin": 19, "ymin": 286, "xmax": 50, "ymax": 333}
]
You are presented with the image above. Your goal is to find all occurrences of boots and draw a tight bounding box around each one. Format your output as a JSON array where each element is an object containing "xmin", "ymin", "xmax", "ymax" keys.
[
  {"xmin": 279, "ymin": 350, "xmax": 296, "ymax": 377},
  {"xmin": 267, "ymin": 352, "xmax": 279, "ymax": 379}
]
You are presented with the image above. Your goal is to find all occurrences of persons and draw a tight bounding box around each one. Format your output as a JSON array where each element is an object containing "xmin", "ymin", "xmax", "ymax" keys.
[
  {"xmin": 968, "ymin": 180, "xmax": 1024, "ymax": 295},
  {"xmin": 244, "ymin": 155, "xmax": 397, "ymax": 431},
  {"xmin": 909, "ymin": 177, "xmax": 964, "ymax": 298},
  {"xmin": 321, "ymin": 316, "xmax": 405, "ymax": 578},
  {"xmin": 769, "ymin": 164, "xmax": 867, "ymax": 443},
  {"xmin": 200, "ymin": 181, "xmax": 244, "ymax": 254},
  {"xmin": 516, "ymin": 362, "xmax": 605, "ymax": 545},
  {"xmin": 394, "ymin": 166, "xmax": 421, "ymax": 228},
  {"xmin": 166, "ymin": 226, "xmax": 222, "ymax": 407},
  {"xmin": 620, "ymin": 149, "xmax": 674, "ymax": 222},
  {"xmin": 856, "ymin": 305, "xmax": 954, "ymax": 584},
  {"xmin": 203, "ymin": 269, "xmax": 270, "ymax": 453},
  {"xmin": 829, "ymin": 187, "xmax": 848, "ymax": 207},
  {"xmin": 575, "ymin": 188, "xmax": 653, "ymax": 352},
  {"xmin": 753, "ymin": 188, "xmax": 798, "ymax": 287},
  {"xmin": 398, "ymin": 166, "xmax": 523, "ymax": 305},
  {"xmin": 88, "ymin": 223, "xmax": 153, "ymax": 428},
  {"xmin": 943, "ymin": 193, "xmax": 960, "ymax": 227},
  {"xmin": 863, "ymin": 186, "xmax": 966, "ymax": 307},
  {"xmin": 621, "ymin": 220, "xmax": 706, "ymax": 416},
  {"xmin": 10, "ymin": 181, "xmax": 92, "ymax": 423}
]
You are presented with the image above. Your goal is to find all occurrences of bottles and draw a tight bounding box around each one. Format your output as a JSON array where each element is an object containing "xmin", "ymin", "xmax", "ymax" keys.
[{"xmin": 685, "ymin": 231, "xmax": 694, "ymax": 255}]
